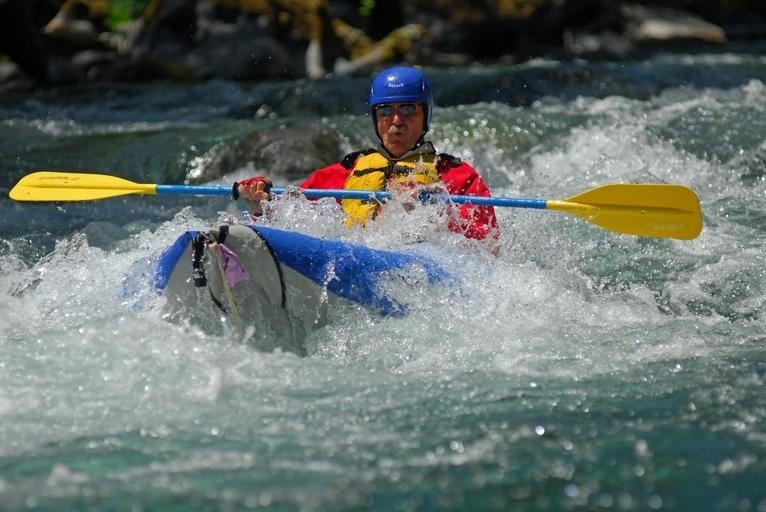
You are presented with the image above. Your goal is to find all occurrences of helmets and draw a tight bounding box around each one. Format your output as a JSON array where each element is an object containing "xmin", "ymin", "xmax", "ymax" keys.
[{"xmin": 369, "ymin": 66, "xmax": 432, "ymax": 132}]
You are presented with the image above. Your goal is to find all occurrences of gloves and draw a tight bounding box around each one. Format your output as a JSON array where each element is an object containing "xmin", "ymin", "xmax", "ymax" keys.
[{"xmin": 238, "ymin": 176, "xmax": 273, "ymax": 201}]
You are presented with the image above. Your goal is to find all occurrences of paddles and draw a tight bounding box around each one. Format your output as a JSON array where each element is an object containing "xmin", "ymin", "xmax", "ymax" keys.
[{"xmin": 9, "ymin": 171, "xmax": 704, "ymax": 240}]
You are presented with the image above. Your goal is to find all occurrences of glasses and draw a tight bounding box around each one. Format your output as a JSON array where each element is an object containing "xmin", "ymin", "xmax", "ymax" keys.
[{"xmin": 375, "ymin": 103, "xmax": 416, "ymax": 116}]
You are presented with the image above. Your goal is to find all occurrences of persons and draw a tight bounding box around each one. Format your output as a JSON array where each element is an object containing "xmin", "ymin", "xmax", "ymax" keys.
[{"xmin": 237, "ymin": 64, "xmax": 500, "ymax": 263}]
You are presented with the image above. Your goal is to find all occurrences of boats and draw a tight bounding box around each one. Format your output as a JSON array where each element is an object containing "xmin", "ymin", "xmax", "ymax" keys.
[{"xmin": 119, "ymin": 225, "xmax": 461, "ymax": 355}]
